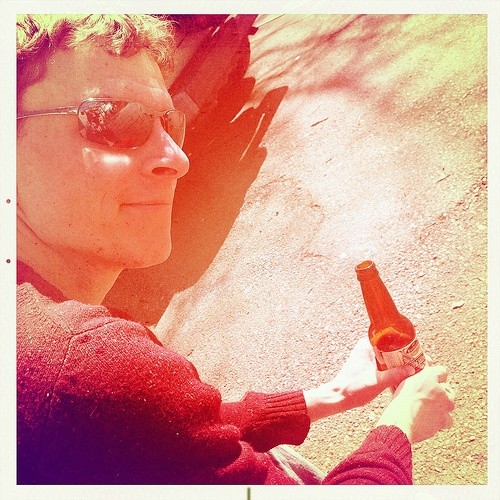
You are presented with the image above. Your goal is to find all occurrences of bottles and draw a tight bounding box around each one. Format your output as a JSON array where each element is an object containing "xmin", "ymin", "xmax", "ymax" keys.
[{"xmin": 354, "ymin": 260, "xmax": 428, "ymax": 396}]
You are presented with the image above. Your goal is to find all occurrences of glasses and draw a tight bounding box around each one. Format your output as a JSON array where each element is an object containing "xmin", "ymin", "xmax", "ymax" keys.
[{"xmin": 18, "ymin": 97, "xmax": 186, "ymax": 151}]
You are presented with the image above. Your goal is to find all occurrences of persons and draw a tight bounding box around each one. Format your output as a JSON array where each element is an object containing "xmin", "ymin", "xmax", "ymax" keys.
[{"xmin": 16, "ymin": 15, "xmax": 457, "ymax": 484}]
